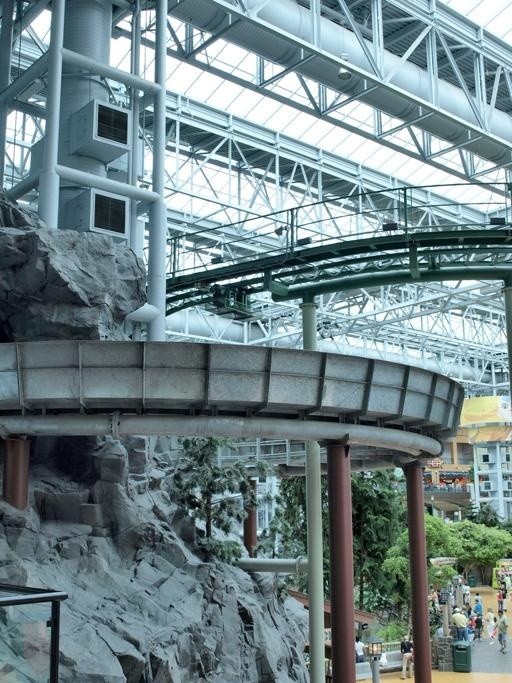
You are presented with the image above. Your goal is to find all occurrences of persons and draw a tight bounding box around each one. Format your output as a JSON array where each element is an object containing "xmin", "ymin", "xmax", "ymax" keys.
[
  {"xmin": 428, "ymin": 580, "xmax": 509, "ymax": 654},
  {"xmin": 355, "ymin": 635, "xmax": 366, "ymax": 663},
  {"xmin": 400, "ymin": 635, "xmax": 413, "ymax": 680}
]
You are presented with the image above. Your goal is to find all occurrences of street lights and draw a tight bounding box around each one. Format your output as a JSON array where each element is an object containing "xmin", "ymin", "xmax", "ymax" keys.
[
  {"xmin": 440, "ymin": 572, "xmax": 464, "ymax": 635},
  {"xmin": 366, "ymin": 634, "xmax": 385, "ymax": 681}
]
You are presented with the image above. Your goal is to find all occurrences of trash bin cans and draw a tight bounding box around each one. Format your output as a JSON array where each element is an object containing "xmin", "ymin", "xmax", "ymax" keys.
[
  {"xmin": 453, "ymin": 640, "xmax": 472, "ymax": 672},
  {"xmin": 468, "ymin": 574, "xmax": 476, "ymax": 587}
]
